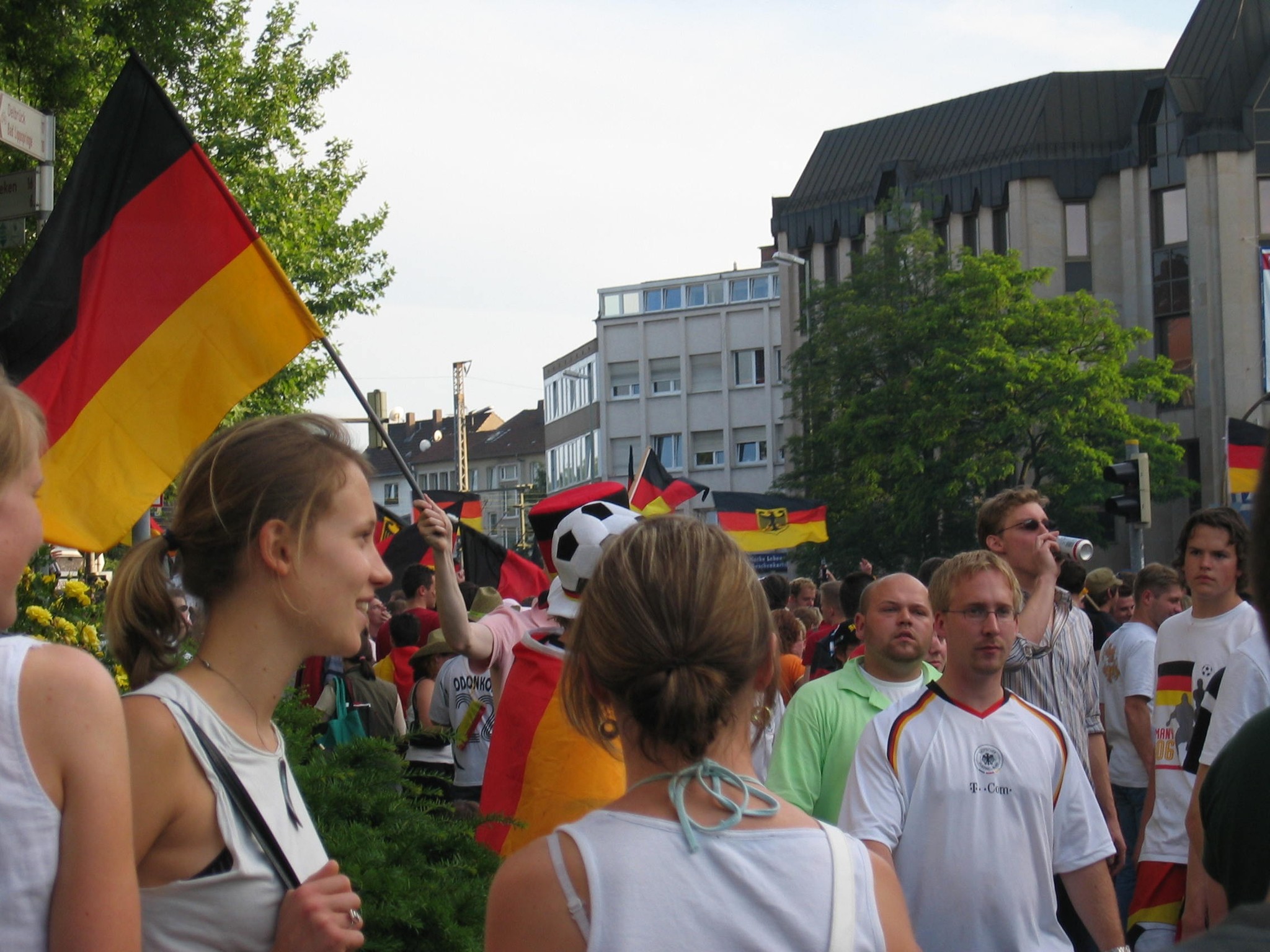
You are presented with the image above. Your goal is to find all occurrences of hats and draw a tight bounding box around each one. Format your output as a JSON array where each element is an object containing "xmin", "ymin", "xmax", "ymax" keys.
[
  {"xmin": 546, "ymin": 499, "xmax": 647, "ymax": 621},
  {"xmin": 467, "ymin": 586, "xmax": 510, "ymax": 622},
  {"xmin": 408, "ymin": 627, "xmax": 454, "ymax": 672},
  {"xmin": 1084, "ymin": 567, "xmax": 1123, "ymax": 594}
]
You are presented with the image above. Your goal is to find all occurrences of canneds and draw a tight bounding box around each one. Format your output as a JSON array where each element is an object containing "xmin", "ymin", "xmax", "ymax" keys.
[{"xmin": 1051, "ymin": 535, "xmax": 1094, "ymax": 563}]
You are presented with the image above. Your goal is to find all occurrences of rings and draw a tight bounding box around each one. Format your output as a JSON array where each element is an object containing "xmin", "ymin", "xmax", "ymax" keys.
[{"xmin": 349, "ymin": 908, "xmax": 361, "ymax": 929}]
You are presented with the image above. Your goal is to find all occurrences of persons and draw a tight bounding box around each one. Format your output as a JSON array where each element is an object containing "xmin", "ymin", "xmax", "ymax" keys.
[
  {"xmin": 751, "ymin": 557, "xmax": 947, "ymax": 827},
  {"xmin": 976, "ymin": 486, "xmax": 1128, "ymax": 952},
  {"xmin": 838, "ymin": 550, "xmax": 1125, "ymax": 952},
  {"xmin": 1056, "ymin": 507, "xmax": 1270, "ymax": 952},
  {"xmin": 0, "ymin": 364, "xmax": 142, "ymax": 952},
  {"xmin": 66, "ymin": 414, "xmax": 394, "ymax": 952},
  {"xmin": 296, "ymin": 482, "xmax": 647, "ymax": 862}
]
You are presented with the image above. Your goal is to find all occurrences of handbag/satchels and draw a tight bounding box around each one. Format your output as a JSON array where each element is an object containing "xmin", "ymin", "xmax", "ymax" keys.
[{"xmin": 311, "ymin": 677, "xmax": 367, "ymax": 753}]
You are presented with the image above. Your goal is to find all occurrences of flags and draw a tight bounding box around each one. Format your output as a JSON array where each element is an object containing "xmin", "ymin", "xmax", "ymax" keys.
[
  {"xmin": 445, "ymin": 509, "xmax": 552, "ymax": 605},
  {"xmin": 370, "ymin": 503, "xmax": 429, "ymax": 605},
  {"xmin": 0, "ymin": 54, "xmax": 324, "ymax": 553},
  {"xmin": 411, "ymin": 489, "xmax": 482, "ymax": 571},
  {"xmin": 1225, "ymin": 417, "xmax": 1270, "ymax": 494},
  {"xmin": 627, "ymin": 446, "xmax": 710, "ymax": 518},
  {"xmin": 712, "ymin": 491, "xmax": 829, "ymax": 553}
]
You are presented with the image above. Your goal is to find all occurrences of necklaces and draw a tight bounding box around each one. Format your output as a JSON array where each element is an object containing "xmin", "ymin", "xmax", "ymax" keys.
[{"xmin": 195, "ymin": 654, "xmax": 303, "ymax": 827}]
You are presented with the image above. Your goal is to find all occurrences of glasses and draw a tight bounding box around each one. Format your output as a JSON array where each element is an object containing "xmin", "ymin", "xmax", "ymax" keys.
[
  {"xmin": 942, "ymin": 607, "xmax": 1021, "ymax": 621},
  {"xmin": 992, "ymin": 518, "xmax": 1057, "ymax": 536}
]
